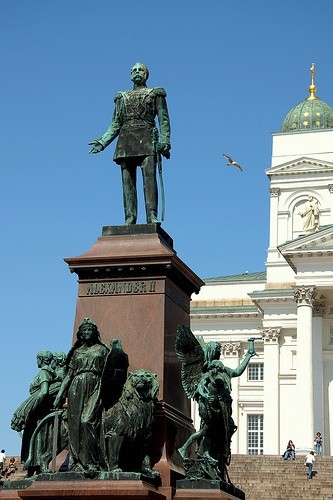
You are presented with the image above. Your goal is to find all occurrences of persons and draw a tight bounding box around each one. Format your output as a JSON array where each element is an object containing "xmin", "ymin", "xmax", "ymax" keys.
[
  {"xmin": 0, "ymin": 449, "xmax": 17, "ymax": 478},
  {"xmin": 304, "ymin": 451, "xmax": 315, "ymax": 478},
  {"xmin": 10, "ymin": 318, "xmax": 256, "ymax": 482},
  {"xmin": 313, "ymin": 432, "xmax": 323, "ymax": 457},
  {"xmin": 86, "ymin": 63, "xmax": 172, "ymax": 225},
  {"xmin": 282, "ymin": 441, "xmax": 295, "ymax": 460}
]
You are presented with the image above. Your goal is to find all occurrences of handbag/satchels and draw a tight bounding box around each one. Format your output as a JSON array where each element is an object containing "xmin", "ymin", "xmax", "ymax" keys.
[{"xmin": 313, "ymin": 441, "xmax": 317, "ymax": 445}]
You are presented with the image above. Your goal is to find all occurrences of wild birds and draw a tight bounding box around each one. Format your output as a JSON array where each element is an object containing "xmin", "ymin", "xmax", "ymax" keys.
[{"xmin": 222, "ymin": 154, "xmax": 243, "ymax": 172}]
